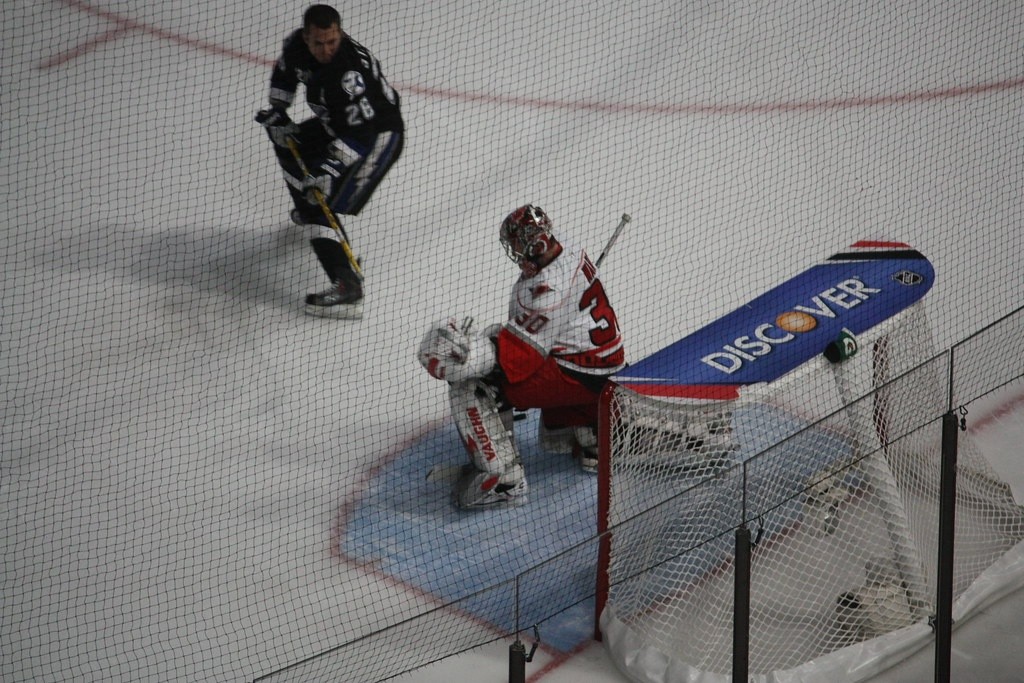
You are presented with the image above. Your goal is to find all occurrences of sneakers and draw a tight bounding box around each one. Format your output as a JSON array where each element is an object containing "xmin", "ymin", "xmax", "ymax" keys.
[{"xmin": 305, "ymin": 258, "xmax": 367, "ymax": 320}]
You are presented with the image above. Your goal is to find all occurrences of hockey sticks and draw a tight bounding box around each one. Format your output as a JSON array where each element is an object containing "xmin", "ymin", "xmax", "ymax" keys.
[
  {"xmin": 285, "ymin": 134, "xmax": 365, "ymax": 283},
  {"xmin": 424, "ymin": 212, "xmax": 634, "ymax": 485}
]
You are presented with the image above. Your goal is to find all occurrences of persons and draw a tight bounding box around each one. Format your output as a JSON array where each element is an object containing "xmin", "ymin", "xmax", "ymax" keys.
[
  {"xmin": 254, "ymin": 4, "xmax": 405, "ymax": 306},
  {"xmin": 421, "ymin": 204, "xmax": 741, "ymax": 509}
]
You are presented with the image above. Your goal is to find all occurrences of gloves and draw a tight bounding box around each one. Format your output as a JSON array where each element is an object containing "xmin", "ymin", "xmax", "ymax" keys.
[
  {"xmin": 301, "ymin": 156, "xmax": 346, "ymax": 205},
  {"xmin": 256, "ymin": 107, "xmax": 303, "ymax": 149}
]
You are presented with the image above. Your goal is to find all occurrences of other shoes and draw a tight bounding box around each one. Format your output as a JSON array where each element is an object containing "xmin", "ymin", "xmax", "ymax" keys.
[{"xmin": 458, "ymin": 465, "xmax": 530, "ymax": 509}]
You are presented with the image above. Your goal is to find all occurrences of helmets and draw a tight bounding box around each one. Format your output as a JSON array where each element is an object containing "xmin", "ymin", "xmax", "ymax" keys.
[{"xmin": 498, "ymin": 204, "xmax": 556, "ymax": 265}]
